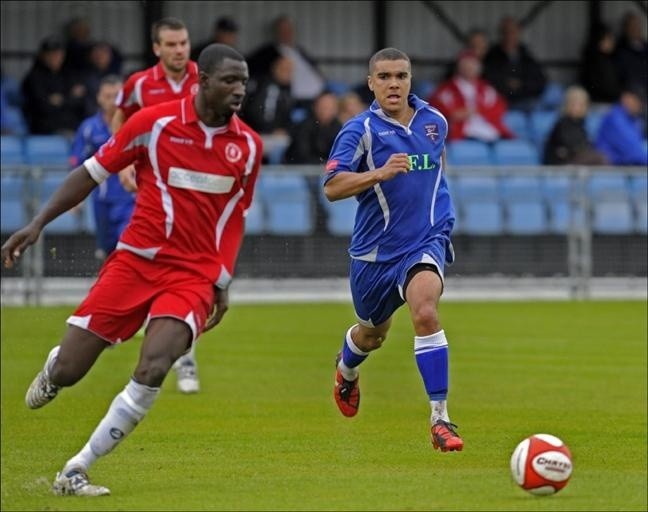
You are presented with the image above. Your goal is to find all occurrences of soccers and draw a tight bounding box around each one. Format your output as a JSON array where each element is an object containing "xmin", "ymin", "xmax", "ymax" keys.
[{"xmin": 510, "ymin": 433, "xmax": 574, "ymax": 497}]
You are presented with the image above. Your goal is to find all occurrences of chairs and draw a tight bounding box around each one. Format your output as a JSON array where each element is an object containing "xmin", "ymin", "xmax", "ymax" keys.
[
  {"xmin": 532, "ymin": 111, "xmax": 559, "ymax": 148},
  {"xmin": 0, "ymin": 93, "xmax": 27, "ymax": 134},
  {"xmin": 82, "ymin": 189, "xmax": 105, "ymax": 273},
  {"xmin": 234, "ymin": 175, "xmax": 261, "ymax": 277},
  {"xmin": 547, "ymin": 178, "xmax": 591, "ymax": 273},
  {"xmin": 32, "ymin": 172, "xmax": 83, "ymax": 274},
  {"xmin": 502, "ymin": 177, "xmax": 546, "ymax": 275},
  {"xmin": 0, "ymin": 136, "xmax": 27, "ymax": 172},
  {"xmin": 456, "ymin": 175, "xmax": 504, "ymax": 276},
  {"xmin": 590, "ymin": 177, "xmax": 635, "ymax": 277},
  {"xmin": 539, "ymin": 86, "xmax": 565, "ymax": 109},
  {"xmin": 504, "ymin": 112, "xmax": 535, "ymax": 144},
  {"xmin": 631, "ymin": 176, "xmax": 648, "ymax": 273},
  {"xmin": 493, "ymin": 139, "xmax": 540, "ymax": 171},
  {"xmin": 453, "ymin": 141, "xmax": 494, "ymax": 173},
  {"xmin": 319, "ymin": 177, "xmax": 361, "ymax": 275},
  {"xmin": 0, "ymin": 171, "xmax": 35, "ymax": 277},
  {"xmin": 29, "ymin": 134, "xmax": 68, "ymax": 170},
  {"xmin": 262, "ymin": 177, "xmax": 315, "ymax": 276}
]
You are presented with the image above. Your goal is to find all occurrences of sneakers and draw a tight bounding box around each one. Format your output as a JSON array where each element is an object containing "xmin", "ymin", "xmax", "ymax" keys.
[
  {"xmin": 430, "ymin": 419, "xmax": 464, "ymax": 452},
  {"xmin": 50, "ymin": 468, "xmax": 111, "ymax": 497},
  {"xmin": 175, "ymin": 358, "xmax": 200, "ymax": 393},
  {"xmin": 24, "ymin": 345, "xmax": 63, "ymax": 410},
  {"xmin": 333, "ymin": 351, "xmax": 360, "ymax": 418}
]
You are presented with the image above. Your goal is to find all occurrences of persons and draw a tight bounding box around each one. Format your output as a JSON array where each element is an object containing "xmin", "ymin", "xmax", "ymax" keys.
[
  {"xmin": 235, "ymin": 54, "xmax": 296, "ymax": 165},
  {"xmin": 338, "ymin": 91, "xmax": 374, "ymax": 127},
  {"xmin": 577, "ymin": 24, "xmax": 624, "ymax": 105},
  {"xmin": 616, "ymin": 12, "xmax": 648, "ymax": 116},
  {"xmin": 593, "ymin": 85, "xmax": 648, "ymax": 166},
  {"xmin": 538, "ymin": 84, "xmax": 612, "ymax": 166},
  {"xmin": 439, "ymin": 32, "xmax": 489, "ymax": 82},
  {"xmin": 56, "ymin": 16, "xmax": 100, "ymax": 73},
  {"xmin": 323, "ymin": 47, "xmax": 464, "ymax": 452},
  {"xmin": 244, "ymin": 13, "xmax": 331, "ymax": 110},
  {"xmin": 479, "ymin": 16, "xmax": 548, "ymax": 116},
  {"xmin": 427, "ymin": 51, "xmax": 517, "ymax": 149},
  {"xmin": 0, "ymin": 43, "xmax": 264, "ymax": 496},
  {"xmin": 189, "ymin": 16, "xmax": 240, "ymax": 62},
  {"xmin": 18, "ymin": 35, "xmax": 86, "ymax": 135},
  {"xmin": 70, "ymin": 42, "xmax": 114, "ymax": 133},
  {"xmin": 112, "ymin": 16, "xmax": 200, "ymax": 394},
  {"xmin": 281, "ymin": 89, "xmax": 344, "ymax": 166},
  {"xmin": 66, "ymin": 76, "xmax": 136, "ymax": 261}
]
